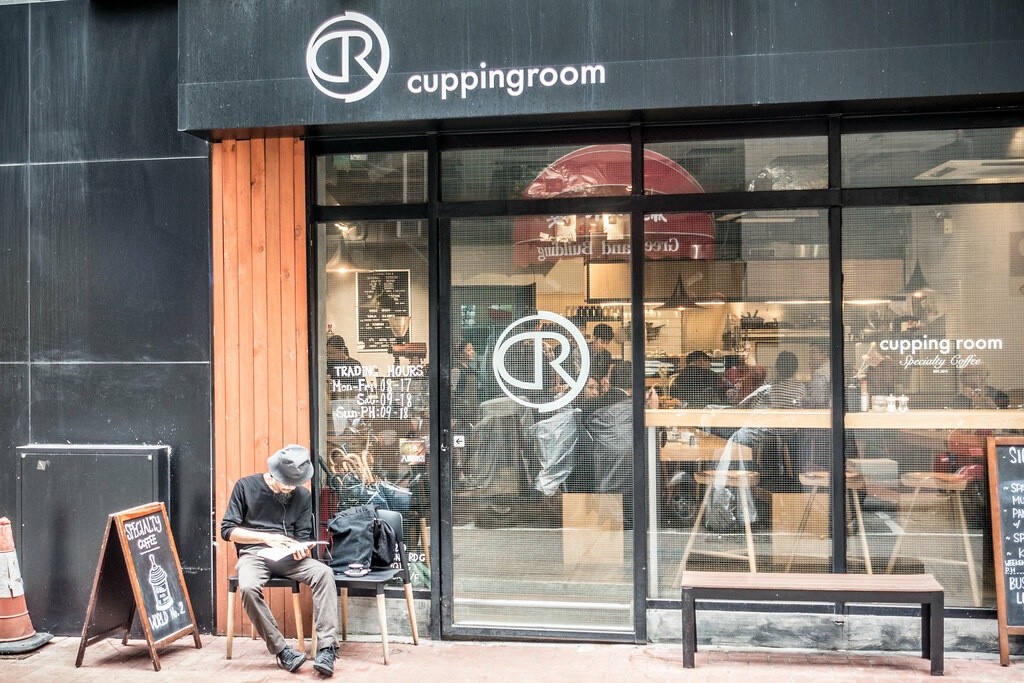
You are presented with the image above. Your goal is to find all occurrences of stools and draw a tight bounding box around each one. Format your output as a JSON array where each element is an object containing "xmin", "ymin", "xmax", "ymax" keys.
[
  {"xmin": 672, "ymin": 471, "xmax": 761, "ymax": 588},
  {"xmin": 886, "ymin": 472, "xmax": 981, "ymax": 607},
  {"xmin": 786, "ymin": 471, "xmax": 873, "ymax": 574}
]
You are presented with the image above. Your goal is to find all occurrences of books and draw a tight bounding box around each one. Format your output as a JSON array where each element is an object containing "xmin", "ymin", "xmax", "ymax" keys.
[{"xmin": 258, "ymin": 541, "xmax": 329, "ymax": 561}]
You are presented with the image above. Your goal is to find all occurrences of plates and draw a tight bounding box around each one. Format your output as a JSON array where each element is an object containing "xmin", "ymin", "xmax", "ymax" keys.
[
  {"xmin": 344, "ymin": 569, "xmax": 372, "ymax": 577},
  {"xmin": 667, "ymin": 438, "xmax": 678, "ymax": 442},
  {"xmin": 678, "ymin": 439, "xmax": 689, "ymax": 444}
]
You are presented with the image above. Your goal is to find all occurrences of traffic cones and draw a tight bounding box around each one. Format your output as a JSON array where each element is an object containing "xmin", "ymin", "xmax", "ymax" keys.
[{"xmin": 0, "ymin": 516, "xmax": 55, "ymax": 655}]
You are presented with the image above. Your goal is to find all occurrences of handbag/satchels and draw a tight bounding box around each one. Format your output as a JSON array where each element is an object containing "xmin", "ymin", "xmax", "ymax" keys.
[{"xmin": 327, "ymin": 512, "xmax": 396, "ymax": 572}]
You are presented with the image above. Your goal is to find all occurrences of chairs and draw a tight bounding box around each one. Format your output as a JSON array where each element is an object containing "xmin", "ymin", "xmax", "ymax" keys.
[
  {"xmin": 227, "ymin": 513, "xmax": 319, "ymax": 660},
  {"xmin": 466, "ymin": 397, "xmax": 634, "ymax": 524},
  {"xmin": 333, "ymin": 509, "xmax": 419, "ymax": 666}
]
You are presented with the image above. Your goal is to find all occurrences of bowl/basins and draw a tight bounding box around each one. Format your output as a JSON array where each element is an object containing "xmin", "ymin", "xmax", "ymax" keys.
[
  {"xmin": 672, "ymin": 433, "xmax": 680, "ymax": 439},
  {"xmin": 681, "ymin": 433, "xmax": 694, "ymax": 440}
]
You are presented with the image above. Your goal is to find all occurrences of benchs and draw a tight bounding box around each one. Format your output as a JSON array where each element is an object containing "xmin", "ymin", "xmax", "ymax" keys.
[
  {"xmin": 680, "ymin": 571, "xmax": 944, "ymax": 677},
  {"xmin": 757, "ymin": 485, "xmax": 829, "ymax": 565}
]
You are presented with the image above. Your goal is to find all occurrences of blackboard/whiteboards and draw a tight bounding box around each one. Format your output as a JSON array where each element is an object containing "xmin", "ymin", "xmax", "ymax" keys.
[
  {"xmin": 986, "ymin": 435, "xmax": 1024, "ymax": 636},
  {"xmin": 84, "ymin": 502, "xmax": 197, "ymax": 649}
]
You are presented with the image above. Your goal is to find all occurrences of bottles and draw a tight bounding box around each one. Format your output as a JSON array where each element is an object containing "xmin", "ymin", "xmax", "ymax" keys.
[
  {"xmin": 148, "ymin": 554, "xmax": 174, "ymax": 611},
  {"xmin": 689, "ymin": 435, "xmax": 695, "ymax": 445},
  {"xmin": 723, "ymin": 314, "xmax": 731, "ymax": 334},
  {"xmin": 654, "ymin": 350, "xmax": 659, "ymax": 356},
  {"xmin": 661, "ymin": 351, "xmax": 666, "ymax": 356},
  {"xmin": 566, "ymin": 306, "xmax": 621, "ymax": 316},
  {"xmin": 326, "ymin": 325, "xmax": 335, "ymax": 340},
  {"xmin": 886, "ymin": 393, "xmax": 910, "ymax": 411},
  {"xmin": 860, "ymin": 381, "xmax": 869, "ymax": 411}
]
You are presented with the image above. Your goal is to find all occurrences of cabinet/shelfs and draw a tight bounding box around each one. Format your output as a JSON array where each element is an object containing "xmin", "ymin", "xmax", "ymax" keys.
[{"xmin": 566, "ymin": 306, "xmax": 625, "ymax": 364}]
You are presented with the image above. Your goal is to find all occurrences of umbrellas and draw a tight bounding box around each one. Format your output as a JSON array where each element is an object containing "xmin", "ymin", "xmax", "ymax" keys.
[{"xmin": 319, "ymin": 448, "xmax": 431, "ymax": 588}]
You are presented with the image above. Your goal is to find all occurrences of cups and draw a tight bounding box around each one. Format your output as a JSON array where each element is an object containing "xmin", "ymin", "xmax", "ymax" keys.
[
  {"xmin": 844, "ymin": 377, "xmax": 860, "ymax": 412},
  {"xmin": 349, "ymin": 564, "xmax": 363, "ymax": 573},
  {"xmin": 863, "ymin": 330, "xmax": 873, "ymax": 335},
  {"xmin": 871, "ymin": 396, "xmax": 886, "ymax": 413}
]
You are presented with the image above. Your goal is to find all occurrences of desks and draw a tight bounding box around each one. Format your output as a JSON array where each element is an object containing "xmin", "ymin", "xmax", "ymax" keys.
[
  {"xmin": 897, "ymin": 429, "xmax": 1013, "ymax": 471},
  {"xmin": 657, "ymin": 426, "xmax": 753, "ymax": 500},
  {"xmin": 645, "ymin": 408, "xmax": 1024, "ymax": 599}
]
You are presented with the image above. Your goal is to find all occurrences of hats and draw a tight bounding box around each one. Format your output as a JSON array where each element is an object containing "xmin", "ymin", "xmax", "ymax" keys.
[{"xmin": 267, "ymin": 445, "xmax": 314, "ymax": 485}]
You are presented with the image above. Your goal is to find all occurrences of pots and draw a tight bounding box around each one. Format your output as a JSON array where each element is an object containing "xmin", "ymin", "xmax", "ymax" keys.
[
  {"xmin": 766, "ymin": 318, "xmax": 790, "ymax": 323},
  {"xmin": 740, "ymin": 310, "xmax": 765, "ymax": 322}
]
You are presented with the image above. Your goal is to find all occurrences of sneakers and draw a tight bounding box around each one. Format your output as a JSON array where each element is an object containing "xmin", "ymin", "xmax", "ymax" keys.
[
  {"xmin": 276, "ymin": 645, "xmax": 307, "ymax": 672},
  {"xmin": 313, "ymin": 645, "xmax": 340, "ymax": 676}
]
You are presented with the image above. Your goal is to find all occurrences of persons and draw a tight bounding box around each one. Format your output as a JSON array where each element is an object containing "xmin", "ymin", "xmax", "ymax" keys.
[
  {"xmin": 499, "ymin": 311, "xmax": 669, "ymax": 528},
  {"xmin": 946, "ymin": 364, "xmax": 1010, "ymax": 407},
  {"xmin": 727, "ymin": 341, "xmax": 766, "ymax": 404},
  {"xmin": 455, "ymin": 336, "xmax": 484, "ymax": 491},
  {"xmin": 755, "ymin": 350, "xmax": 805, "ymax": 477},
  {"xmin": 804, "ymin": 341, "xmax": 832, "ymax": 407},
  {"xmin": 671, "ymin": 351, "xmax": 729, "ymax": 409},
  {"xmin": 220, "ymin": 445, "xmax": 340, "ymax": 676},
  {"xmin": 327, "ymin": 336, "xmax": 363, "ymax": 399}
]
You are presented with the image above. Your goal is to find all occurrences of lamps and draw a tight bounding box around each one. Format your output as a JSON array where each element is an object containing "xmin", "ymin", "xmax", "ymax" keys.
[
  {"xmin": 654, "ymin": 273, "xmax": 706, "ymax": 311},
  {"xmin": 927, "ymin": 206, "xmax": 947, "ymax": 220},
  {"xmin": 326, "ymin": 235, "xmax": 366, "ymax": 274},
  {"xmin": 898, "ymin": 207, "xmax": 936, "ymax": 298}
]
